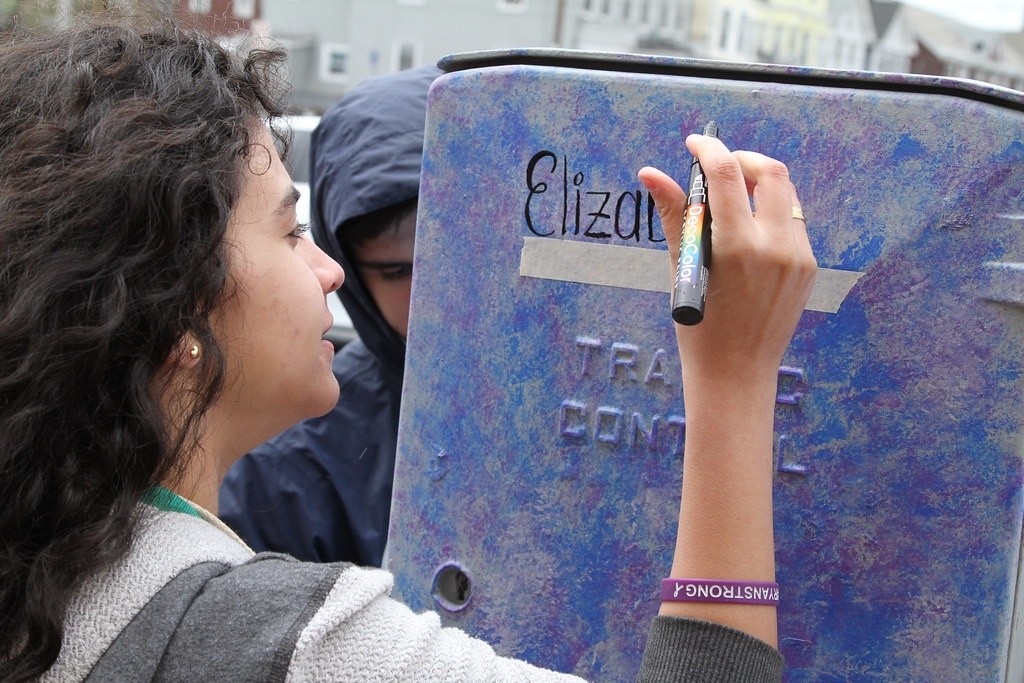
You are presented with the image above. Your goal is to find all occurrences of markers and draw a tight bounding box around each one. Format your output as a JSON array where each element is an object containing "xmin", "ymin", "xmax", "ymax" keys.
[{"xmin": 669, "ymin": 120, "xmax": 721, "ymax": 326}]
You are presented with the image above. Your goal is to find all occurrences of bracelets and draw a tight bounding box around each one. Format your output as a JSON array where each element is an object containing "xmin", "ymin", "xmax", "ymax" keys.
[{"xmin": 659, "ymin": 577, "xmax": 780, "ymax": 606}]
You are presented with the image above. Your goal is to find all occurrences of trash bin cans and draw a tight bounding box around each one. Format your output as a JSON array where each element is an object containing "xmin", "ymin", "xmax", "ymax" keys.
[{"xmin": 382, "ymin": 42, "xmax": 1024, "ymax": 683}]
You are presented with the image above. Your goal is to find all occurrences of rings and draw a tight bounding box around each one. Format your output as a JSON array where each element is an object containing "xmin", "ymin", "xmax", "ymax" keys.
[{"xmin": 792, "ymin": 206, "xmax": 806, "ymax": 222}]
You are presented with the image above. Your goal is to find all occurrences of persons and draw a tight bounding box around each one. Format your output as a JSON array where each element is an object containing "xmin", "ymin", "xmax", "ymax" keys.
[
  {"xmin": 0, "ymin": 21, "xmax": 820, "ymax": 683},
  {"xmin": 218, "ymin": 65, "xmax": 446, "ymax": 569}
]
